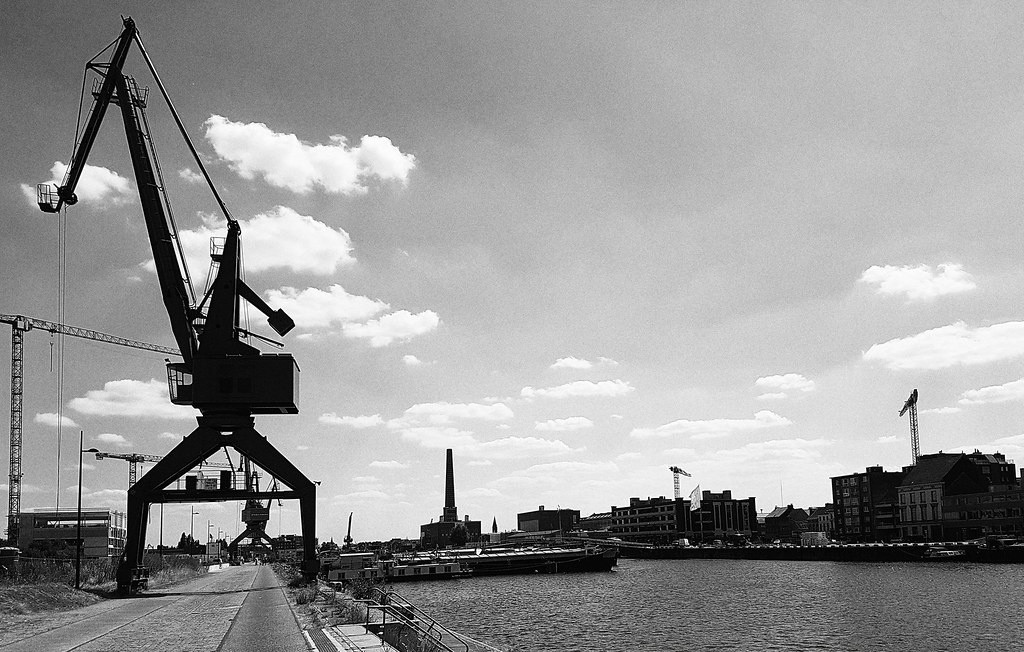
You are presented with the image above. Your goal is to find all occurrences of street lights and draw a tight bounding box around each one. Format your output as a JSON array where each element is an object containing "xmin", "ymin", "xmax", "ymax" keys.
[
  {"xmin": 218, "ymin": 526, "xmax": 224, "ymax": 563},
  {"xmin": 75, "ymin": 430, "xmax": 100, "ymax": 587},
  {"xmin": 190, "ymin": 505, "xmax": 200, "ymax": 557},
  {"xmin": 207, "ymin": 520, "xmax": 214, "ymax": 560}
]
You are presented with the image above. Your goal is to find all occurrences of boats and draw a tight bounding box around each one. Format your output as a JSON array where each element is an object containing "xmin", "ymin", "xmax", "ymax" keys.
[
  {"xmin": 975, "ymin": 536, "xmax": 1024, "ymax": 562},
  {"xmin": 326, "ymin": 541, "xmax": 619, "ymax": 584},
  {"xmin": 929, "ymin": 550, "xmax": 968, "ymax": 561}
]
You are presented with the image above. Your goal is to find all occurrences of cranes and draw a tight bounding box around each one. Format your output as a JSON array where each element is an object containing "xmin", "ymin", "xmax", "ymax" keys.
[
  {"xmin": 36, "ymin": 14, "xmax": 321, "ymax": 589},
  {"xmin": 897, "ymin": 388, "xmax": 921, "ymax": 466},
  {"xmin": 94, "ymin": 450, "xmax": 237, "ymax": 490},
  {"xmin": 669, "ymin": 465, "xmax": 692, "ymax": 499},
  {"xmin": 226, "ymin": 452, "xmax": 281, "ymax": 567},
  {"xmin": 1, "ymin": 312, "xmax": 186, "ymax": 546}
]
